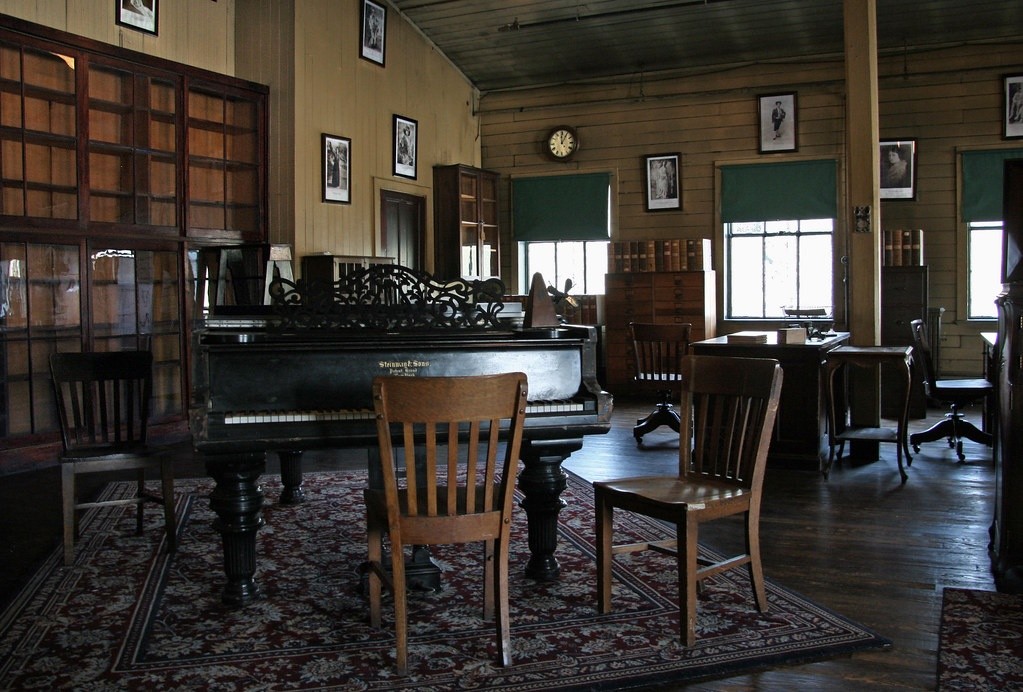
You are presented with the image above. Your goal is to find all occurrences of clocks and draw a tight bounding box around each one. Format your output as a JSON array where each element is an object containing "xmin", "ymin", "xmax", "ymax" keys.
[{"xmin": 546, "ymin": 125, "xmax": 580, "ymax": 162}]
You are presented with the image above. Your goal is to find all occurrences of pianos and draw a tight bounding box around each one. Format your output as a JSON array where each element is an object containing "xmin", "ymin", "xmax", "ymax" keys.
[{"xmin": 191, "ymin": 260, "xmax": 616, "ymax": 607}]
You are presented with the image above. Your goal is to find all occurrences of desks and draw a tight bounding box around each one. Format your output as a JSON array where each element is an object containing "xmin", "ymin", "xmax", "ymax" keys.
[
  {"xmin": 823, "ymin": 346, "xmax": 914, "ymax": 480},
  {"xmin": 979, "ymin": 330, "xmax": 999, "ymax": 446}
]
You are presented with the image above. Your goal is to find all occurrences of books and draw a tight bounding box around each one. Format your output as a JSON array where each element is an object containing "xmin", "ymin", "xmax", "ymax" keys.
[
  {"xmin": 608, "ymin": 238, "xmax": 712, "ymax": 271},
  {"xmin": 882, "ymin": 229, "xmax": 923, "ymax": 266}
]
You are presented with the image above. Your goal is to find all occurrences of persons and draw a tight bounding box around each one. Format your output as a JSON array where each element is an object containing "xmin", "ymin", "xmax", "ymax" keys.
[
  {"xmin": 887, "ymin": 147, "xmax": 908, "ymax": 186},
  {"xmin": 370, "ymin": 9, "xmax": 380, "ymax": 46},
  {"xmin": 1009, "ymin": 88, "xmax": 1023, "ymax": 122},
  {"xmin": 332, "ymin": 146, "xmax": 342, "ymax": 186},
  {"xmin": 656, "ymin": 160, "xmax": 668, "ymax": 199},
  {"xmin": 399, "ymin": 126, "xmax": 413, "ymax": 165},
  {"xmin": 772, "ymin": 101, "xmax": 786, "ymax": 140}
]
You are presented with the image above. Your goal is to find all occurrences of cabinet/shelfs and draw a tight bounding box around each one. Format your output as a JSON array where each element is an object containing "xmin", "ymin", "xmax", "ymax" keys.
[
  {"xmin": 879, "ymin": 265, "xmax": 931, "ymax": 420},
  {"xmin": 603, "ymin": 271, "xmax": 717, "ymax": 399},
  {"xmin": 302, "ymin": 255, "xmax": 394, "ymax": 308},
  {"xmin": 432, "ymin": 164, "xmax": 501, "ymax": 286},
  {"xmin": 0, "ymin": 12, "xmax": 270, "ymax": 468},
  {"xmin": 688, "ymin": 331, "xmax": 850, "ymax": 474}
]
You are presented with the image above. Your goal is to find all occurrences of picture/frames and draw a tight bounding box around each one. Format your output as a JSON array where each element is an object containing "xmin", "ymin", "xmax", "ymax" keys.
[
  {"xmin": 756, "ymin": 91, "xmax": 799, "ymax": 154},
  {"xmin": 644, "ymin": 152, "xmax": 684, "ymax": 213},
  {"xmin": 1002, "ymin": 72, "xmax": 1023, "ymax": 140},
  {"xmin": 878, "ymin": 137, "xmax": 919, "ymax": 202},
  {"xmin": 358, "ymin": 0, "xmax": 387, "ymax": 68},
  {"xmin": 322, "ymin": 132, "xmax": 352, "ymax": 204},
  {"xmin": 115, "ymin": 0, "xmax": 160, "ymax": 38},
  {"xmin": 393, "ymin": 114, "xmax": 418, "ymax": 181}
]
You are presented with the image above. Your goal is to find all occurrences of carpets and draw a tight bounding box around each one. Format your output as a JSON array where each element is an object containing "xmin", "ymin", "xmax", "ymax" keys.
[
  {"xmin": 0, "ymin": 472, "xmax": 895, "ymax": 692},
  {"xmin": 938, "ymin": 586, "xmax": 1023, "ymax": 692}
]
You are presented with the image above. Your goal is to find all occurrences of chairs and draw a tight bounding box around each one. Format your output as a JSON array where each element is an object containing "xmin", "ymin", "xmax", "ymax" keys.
[
  {"xmin": 361, "ymin": 370, "xmax": 531, "ymax": 675},
  {"xmin": 47, "ymin": 346, "xmax": 178, "ymax": 566},
  {"xmin": 909, "ymin": 317, "xmax": 994, "ymax": 463},
  {"xmin": 592, "ymin": 352, "xmax": 781, "ymax": 640},
  {"xmin": 628, "ymin": 321, "xmax": 694, "ymax": 445}
]
